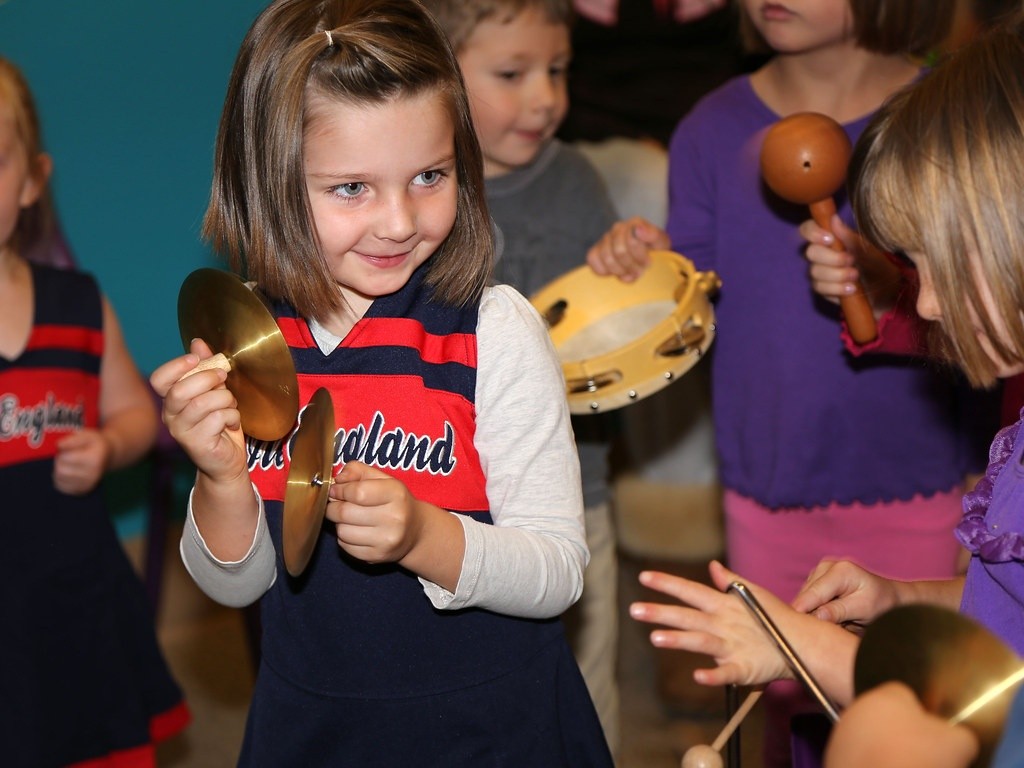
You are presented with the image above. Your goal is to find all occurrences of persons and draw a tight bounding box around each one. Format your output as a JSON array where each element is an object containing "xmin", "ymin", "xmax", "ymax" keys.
[
  {"xmin": 148, "ymin": 0, "xmax": 619, "ymax": 768},
  {"xmin": 0, "ymin": 0, "xmax": 1024, "ymax": 768}
]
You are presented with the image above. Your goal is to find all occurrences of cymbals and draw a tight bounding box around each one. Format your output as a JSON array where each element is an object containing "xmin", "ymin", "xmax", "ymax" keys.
[
  {"xmin": 526, "ymin": 247, "xmax": 725, "ymax": 415},
  {"xmin": 852, "ymin": 602, "xmax": 1024, "ymax": 766},
  {"xmin": 285, "ymin": 385, "xmax": 334, "ymax": 576},
  {"xmin": 178, "ymin": 267, "xmax": 297, "ymax": 442}
]
